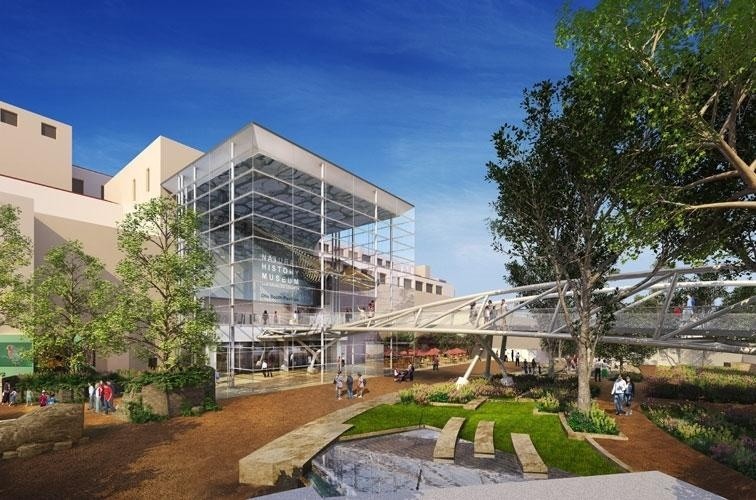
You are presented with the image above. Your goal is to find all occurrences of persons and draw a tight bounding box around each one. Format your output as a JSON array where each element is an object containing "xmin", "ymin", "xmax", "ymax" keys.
[
  {"xmin": 38, "ymin": 389, "xmax": 55, "ymax": 407},
  {"xmin": 434, "ymin": 355, "xmax": 439, "ymax": 371},
  {"xmin": 273, "ymin": 311, "xmax": 278, "ymax": 323},
  {"xmin": 431, "ymin": 355, "xmax": 435, "ymax": 370},
  {"xmin": 609, "ymin": 373, "xmax": 627, "ymax": 416},
  {"xmin": 673, "ymin": 304, "xmax": 682, "ymax": 328},
  {"xmin": 344, "ymin": 310, "xmax": 349, "ymax": 325},
  {"xmin": 86, "ymin": 379, "xmax": 115, "ymax": 415},
  {"xmin": 265, "ymin": 359, "xmax": 273, "ymax": 377},
  {"xmin": 25, "ymin": 388, "xmax": 34, "ymax": 407},
  {"xmin": 601, "ymin": 360, "xmax": 611, "ymax": 380},
  {"xmin": 593, "ymin": 358, "xmax": 602, "ymax": 383},
  {"xmin": 340, "ymin": 357, "xmax": 345, "ymax": 370},
  {"xmin": 622, "ymin": 375, "xmax": 635, "ymax": 416},
  {"xmin": 353, "ymin": 374, "xmax": 365, "ymax": 399},
  {"xmin": 260, "ymin": 360, "xmax": 267, "ymax": 376},
  {"xmin": 260, "ymin": 310, "xmax": 269, "ymax": 325},
  {"xmin": 357, "ymin": 306, "xmax": 366, "ymax": 320},
  {"xmin": 333, "ymin": 370, "xmax": 344, "ymax": 401},
  {"xmin": 503, "ymin": 348, "xmax": 537, "ymax": 375},
  {"xmin": 7, "ymin": 388, "xmax": 17, "ymax": 408},
  {"xmin": 467, "ymin": 297, "xmax": 510, "ymax": 331},
  {"xmin": 391, "ymin": 360, "xmax": 414, "ymax": 382},
  {"xmin": 0, "ymin": 379, "xmax": 10, "ymax": 407},
  {"xmin": 345, "ymin": 371, "xmax": 354, "ymax": 400},
  {"xmin": 335, "ymin": 355, "xmax": 340, "ymax": 371}
]
